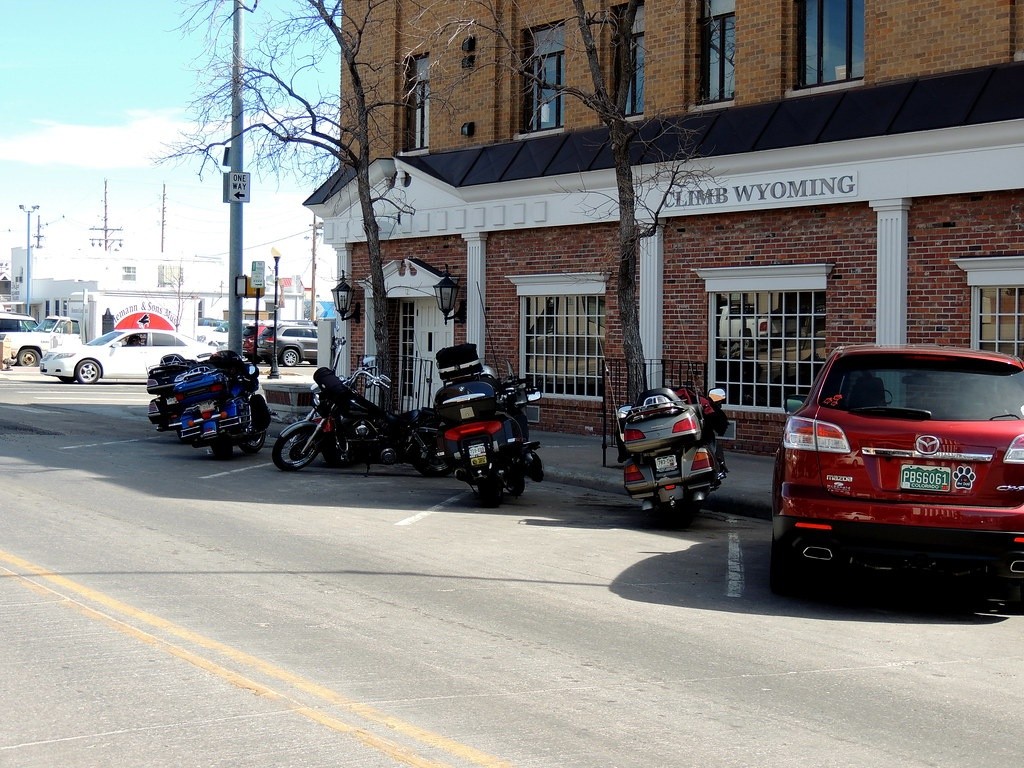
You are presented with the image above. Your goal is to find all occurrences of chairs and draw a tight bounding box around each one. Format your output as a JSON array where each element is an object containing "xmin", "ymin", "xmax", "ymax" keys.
[{"xmin": 849, "ymin": 377, "xmax": 887, "ymax": 407}]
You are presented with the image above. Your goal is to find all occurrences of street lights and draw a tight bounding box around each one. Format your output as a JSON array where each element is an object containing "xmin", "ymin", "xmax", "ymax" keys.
[
  {"xmin": 19, "ymin": 204, "xmax": 40, "ymax": 314},
  {"xmin": 268, "ymin": 244, "xmax": 283, "ymax": 380}
]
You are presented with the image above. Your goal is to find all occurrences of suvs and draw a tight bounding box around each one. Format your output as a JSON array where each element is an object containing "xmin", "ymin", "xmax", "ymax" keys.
[{"xmin": 243, "ymin": 320, "xmax": 318, "ymax": 367}]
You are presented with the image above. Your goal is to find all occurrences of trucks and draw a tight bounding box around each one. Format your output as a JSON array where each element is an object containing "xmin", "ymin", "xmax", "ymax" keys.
[{"xmin": 0, "ymin": 291, "xmax": 202, "ymax": 367}]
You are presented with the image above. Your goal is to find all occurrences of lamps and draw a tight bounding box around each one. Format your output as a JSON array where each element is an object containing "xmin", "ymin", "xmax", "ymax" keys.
[
  {"xmin": 433, "ymin": 263, "xmax": 466, "ymax": 326},
  {"xmin": 331, "ymin": 270, "xmax": 360, "ymax": 324}
]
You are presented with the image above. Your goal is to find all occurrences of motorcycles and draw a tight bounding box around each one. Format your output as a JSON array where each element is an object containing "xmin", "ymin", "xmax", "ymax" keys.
[
  {"xmin": 147, "ymin": 350, "xmax": 271, "ymax": 458},
  {"xmin": 613, "ymin": 385, "xmax": 728, "ymax": 526},
  {"xmin": 433, "ymin": 343, "xmax": 544, "ymax": 508},
  {"xmin": 271, "ymin": 338, "xmax": 458, "ymax": 476}
]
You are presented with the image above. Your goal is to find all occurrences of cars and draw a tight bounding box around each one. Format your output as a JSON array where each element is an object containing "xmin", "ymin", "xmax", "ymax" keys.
[
  {"xmin": 39, "ymin": 329, "xmax": 219, "ymax": 385},
  {"xmin": 771, "ymin": 345, "xmax": 1024, "ymax": 597}
]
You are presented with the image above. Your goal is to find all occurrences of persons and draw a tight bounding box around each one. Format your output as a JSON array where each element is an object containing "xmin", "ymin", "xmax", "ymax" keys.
[{"xmin": 131, "ymin": 336, "xmax": 141, "ymax": 346}]
